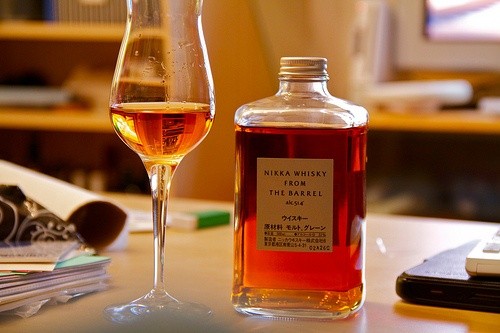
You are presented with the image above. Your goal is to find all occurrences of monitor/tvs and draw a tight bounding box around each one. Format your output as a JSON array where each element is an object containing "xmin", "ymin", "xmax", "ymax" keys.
[{"xmin": 394, "ymin": 0, "xmax": 500, "ymax": 77}]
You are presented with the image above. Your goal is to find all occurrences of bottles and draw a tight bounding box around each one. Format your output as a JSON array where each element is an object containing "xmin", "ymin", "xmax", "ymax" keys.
[{"xmin": 231, "ymin": 58, "xmax": 368, "ymax": 322}]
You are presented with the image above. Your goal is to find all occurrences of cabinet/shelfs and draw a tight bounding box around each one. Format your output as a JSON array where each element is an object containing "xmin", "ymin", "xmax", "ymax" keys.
[{"xmin": 1, "ymin": 0, "xmax": 174, "ymax": 193}]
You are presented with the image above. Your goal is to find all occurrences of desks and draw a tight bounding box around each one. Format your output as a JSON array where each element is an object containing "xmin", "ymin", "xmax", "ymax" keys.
[
  {"xmin": 0, "ymin": 190, "xmax": 500, "ymax": 333},
  {"xmin": 366, "ymin": 63, "xmax": 500, "ymax": 225}
]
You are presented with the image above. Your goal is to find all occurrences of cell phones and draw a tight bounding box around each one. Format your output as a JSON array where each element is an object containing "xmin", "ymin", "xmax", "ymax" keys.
[{"xmin": 395, "ymin": 238, "xmax": 500, "ymax": 312}]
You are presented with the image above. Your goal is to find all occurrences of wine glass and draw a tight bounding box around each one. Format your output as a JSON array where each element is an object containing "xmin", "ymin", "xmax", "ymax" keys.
[{"xmin": 104, "ymin": 0, "xmax": 217, "ymax": 327}]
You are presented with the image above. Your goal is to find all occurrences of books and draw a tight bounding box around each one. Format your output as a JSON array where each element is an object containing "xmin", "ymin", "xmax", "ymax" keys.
[{"xmin": 0, "ymin": 242, "xmax": 112, "ymax": 312}]
